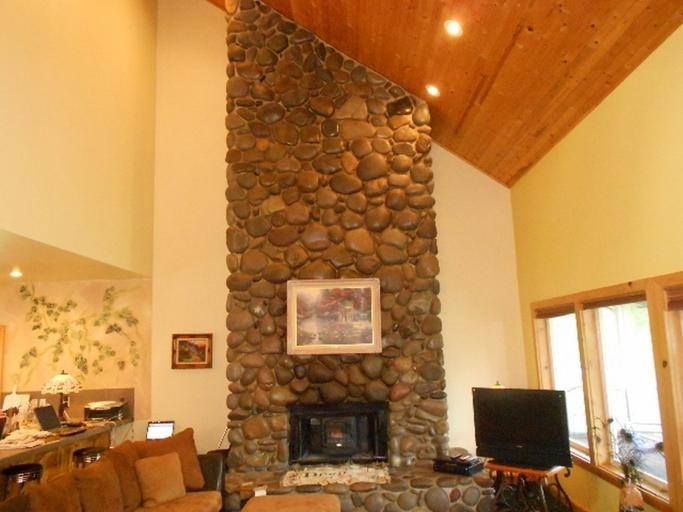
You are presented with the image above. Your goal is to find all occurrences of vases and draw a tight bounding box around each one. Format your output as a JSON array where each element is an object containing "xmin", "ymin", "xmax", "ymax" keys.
[{"xmin": 619, "ymin": 477, "xmax": 645, "ymax": 512}]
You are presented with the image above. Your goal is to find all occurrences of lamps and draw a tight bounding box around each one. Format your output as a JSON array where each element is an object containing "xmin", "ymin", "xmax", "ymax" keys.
[{"xmin": 40, "ymin": 369, "xmax": 82, "ymax": 417}]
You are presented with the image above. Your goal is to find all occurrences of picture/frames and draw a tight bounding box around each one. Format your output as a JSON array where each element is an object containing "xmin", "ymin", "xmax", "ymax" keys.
[
  {"xmin": 286, "ymin": 278, "xmax": 383, "ymax": 355},
  {"xmin": 171, "ymin": 333, "xmax": 212, "ymax": 369}
]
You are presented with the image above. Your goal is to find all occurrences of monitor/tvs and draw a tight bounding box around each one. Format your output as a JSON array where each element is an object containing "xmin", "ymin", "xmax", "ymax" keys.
[
  {"xmin": 146, "ymin": 421, "xmax": 174, "ymax": 440},
  {"xmin": 472, "ymin": 386, "xmax": 573, "ymax": 469}
]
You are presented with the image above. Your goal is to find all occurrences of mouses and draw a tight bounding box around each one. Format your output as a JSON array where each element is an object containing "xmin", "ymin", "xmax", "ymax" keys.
[{"xmin": 68, "ymin": 421, "xmax": 81, "ymax": 427}]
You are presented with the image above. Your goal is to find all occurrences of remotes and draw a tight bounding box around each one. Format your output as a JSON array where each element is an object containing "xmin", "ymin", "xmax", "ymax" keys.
[
  {"xmin": 460, "ymin": 455, "xmax": 470, "ymax": 460},
  {"xmin": 450, "ymin": 453, "xmax": 463, "ymax": 460}
]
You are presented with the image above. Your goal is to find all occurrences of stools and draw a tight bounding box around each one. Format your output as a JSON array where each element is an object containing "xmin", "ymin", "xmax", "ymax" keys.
[
  {"xmin": 71, "ymin": 446, "xmax": 105, "ymax": 470},
  {"xmin": 240, "ymin": 493, "xmax": 341, "ymax": 512},
  {"xmin": 1, "ymin": 464, "xmax": 43, "ymax": 503}
]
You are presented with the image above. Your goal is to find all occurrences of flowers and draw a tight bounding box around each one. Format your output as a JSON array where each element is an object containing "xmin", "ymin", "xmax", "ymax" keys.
[{"xmin": 592, "ymin": 416, "xmax": 665, "ymax": 482}]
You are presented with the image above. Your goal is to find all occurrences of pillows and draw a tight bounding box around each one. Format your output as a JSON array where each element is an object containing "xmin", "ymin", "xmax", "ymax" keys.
[
  {"xmin": 72, "ymin": 455, "xmax": 125, "ymax": 512},
  {"xmin": 133, "ymin": 427, "xmax": 206, "ymax": 491},
  {"xmin": 0, "ymin": 495, "xmax": 26, "ymax": 512},
  {"xmin": 101, "ymin": 439, "xmax": 142, "ymax": 510},
  {"xmin": 135, "ymin": 452, "xmax": 186, "ymax": 507},
  {"xmin": 23, "ymin": 473, "xmax": 82, "ymax": 512}
]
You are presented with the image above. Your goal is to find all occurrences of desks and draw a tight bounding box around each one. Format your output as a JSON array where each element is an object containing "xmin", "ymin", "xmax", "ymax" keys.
[{"xmin": 484, "ymin": 459, "xmax": 572, "ymax": 511}]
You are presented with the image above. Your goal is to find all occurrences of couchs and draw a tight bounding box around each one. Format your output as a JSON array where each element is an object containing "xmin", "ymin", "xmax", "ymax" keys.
[{"xmin": 136, "ymin": 451, "xmax": 226, "ymax": 512}]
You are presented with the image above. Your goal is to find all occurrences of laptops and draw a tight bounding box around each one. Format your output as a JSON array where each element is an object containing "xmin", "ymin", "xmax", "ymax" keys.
[{"xmin": 34, "ymin": 405, "xmax": 86, "ymax": 436}]
